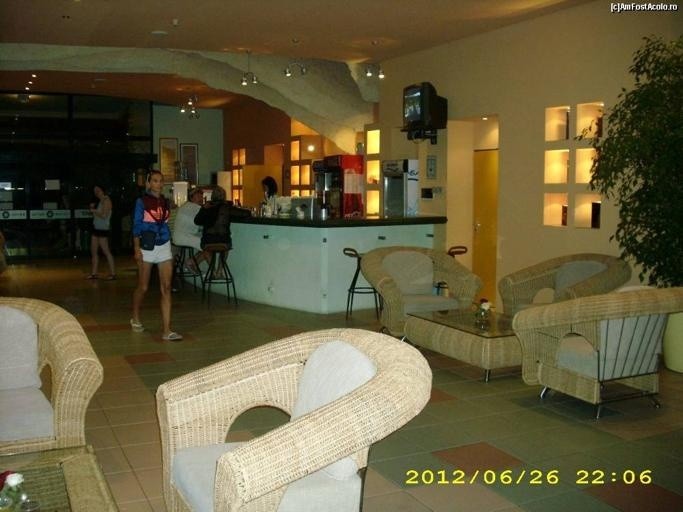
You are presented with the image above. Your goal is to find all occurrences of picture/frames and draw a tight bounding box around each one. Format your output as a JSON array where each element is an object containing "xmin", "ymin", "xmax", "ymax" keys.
[
  {"xmin": 178, "ymin": 142, "xmax": 199, "ymax": 186},
  {"xmin": 158, "ymin": 137, "xmax": 178, "ymax": 184}
]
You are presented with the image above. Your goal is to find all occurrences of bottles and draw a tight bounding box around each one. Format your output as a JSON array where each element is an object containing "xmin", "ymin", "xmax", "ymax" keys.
[
  {"xmin": 178, "ymin": 160, "xmax": 184, "ymax": 181},
  {"xmin": 234, "ymin": 199, "xmax": 241, "ymax": 209}
]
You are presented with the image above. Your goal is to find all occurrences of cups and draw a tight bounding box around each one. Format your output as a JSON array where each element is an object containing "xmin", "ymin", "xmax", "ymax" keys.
[
  {"xmin": 0, "ymin": 493, "xmax": 41, "ymax": 512},
  {"xmin": 250, "ymin": 201, "xmax": 306, "ymax": 221}
]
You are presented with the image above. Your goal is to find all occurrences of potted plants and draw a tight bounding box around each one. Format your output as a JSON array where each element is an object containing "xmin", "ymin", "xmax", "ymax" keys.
[{"xmin": 572, "ymin": 31, "xmax": 683, "ymax": 381}]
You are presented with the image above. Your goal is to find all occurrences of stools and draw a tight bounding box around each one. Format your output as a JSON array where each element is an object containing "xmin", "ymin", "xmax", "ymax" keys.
[
  {"xmin": 434, "ymin": 244, "xmax": 469, "ymax": 298},
  {"xmin": 341, "ymin": 243, "xmax": 381, "ymax": 322},
  {"xmin": 170, "ymin": 241, "xmax": 204, "ymax": 294},
  {"xmin": 200, "ymin": 244, "xmax": 237, "ymax": 310}
]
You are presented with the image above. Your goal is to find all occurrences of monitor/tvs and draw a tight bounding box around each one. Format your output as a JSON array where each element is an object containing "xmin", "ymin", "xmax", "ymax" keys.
[{"xmin": 403, "ymin": 82, "xmax": 447, "ymax": 129}]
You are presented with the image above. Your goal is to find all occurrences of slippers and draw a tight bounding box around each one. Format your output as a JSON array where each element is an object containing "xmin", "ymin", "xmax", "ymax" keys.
[
  {"xmin": 161, "ymin": 332, "xmax": 182, "ymax": 340},
  {"xmin": 130, "ymin": 318, "xmax": 144, "ymax": 333}
]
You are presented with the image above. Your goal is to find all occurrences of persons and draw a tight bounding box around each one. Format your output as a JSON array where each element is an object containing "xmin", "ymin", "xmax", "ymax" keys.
[
  {"xmin": 172, "ymin": 189, "xmax": 203, "ymax": 275},
  {"xmin": 130, "ymin": 171, "xmax": 183, "ymax": 340},
  {"xmin": 195, "ymin": 187, "xmax": 251, "ymax": 279},
  {"xmin": 85, "ymin": 185, "xmax": 118, "ymax": 280},
  {"xmin": 261, "ymin": 176, "xmax": 277, "ymax": 207}
]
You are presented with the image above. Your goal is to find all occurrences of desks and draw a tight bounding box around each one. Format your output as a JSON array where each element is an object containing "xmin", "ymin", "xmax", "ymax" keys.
[
  {"xmin": 398, "ymin": 307, "xmax": 521, "ymax": 387},
  {"xmin": 0, "ymin": 438, "xmax": 119, "ymax": 512}
]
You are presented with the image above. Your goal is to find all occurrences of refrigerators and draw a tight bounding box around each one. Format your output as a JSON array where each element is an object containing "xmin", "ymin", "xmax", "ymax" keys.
[
  {"xmin": 311, "ymin": 154, "xmax": 364, "ymax": 218},
  {"xmin": 382, "ymin": 159, "xmax": 419, "ymax": 218}
]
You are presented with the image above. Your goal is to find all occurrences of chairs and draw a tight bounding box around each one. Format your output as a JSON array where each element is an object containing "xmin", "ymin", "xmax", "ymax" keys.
[
  {"xmin": 151, "ymin": 324, "xmax": 435, "ymax": 511},
  {"xmin": 495, "ymin": 251, "xmax": 631, "ymax": 325},
  {"xmin": 0, "ymin": 293, "xmax": 106, "ymax": 460},
  {"xmin": 358, "ymin": 245, "xmax": 484, "ymax": 337},
  {"xmin": 510, "ymin": 283, "xmax": 682, "ymax": 420}
]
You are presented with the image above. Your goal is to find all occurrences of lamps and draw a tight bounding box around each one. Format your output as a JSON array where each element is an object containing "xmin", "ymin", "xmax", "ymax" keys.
[
  {"xmin": 364, "ymin": 39, "xmax": 387, "ymax": 80},
  {"xmin": 239, "ymin": 45, "xmax": 259, "ymax": 88},
  {"xmin": 282, "ymin": 35, "xmax": 311, "ymax": 80},
  {"xmin": 179, "ymin": 91, "xmax": 199, "ymax": 115}
]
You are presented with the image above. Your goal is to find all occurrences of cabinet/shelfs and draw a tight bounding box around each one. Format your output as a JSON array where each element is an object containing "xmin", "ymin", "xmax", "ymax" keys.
[
  {"xmin": 229, "ymin": 143, "xmax": 262, "ymax": 207},
  {"xmin": 360, "ymin": 122, "xmax": 418, "ymax": 217},
  {"xmin": 289, "ymin": 134, "xmax": 321, "ymax": 198},
  {"xmin": 541, "ymin": 97, "xmax": 605, "ymax": 230}
]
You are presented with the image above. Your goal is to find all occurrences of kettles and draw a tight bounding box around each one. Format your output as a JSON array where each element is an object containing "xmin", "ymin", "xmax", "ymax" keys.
[{"xmin": 319, "ymin": 204, "xmax": 330, "ymax": 222}]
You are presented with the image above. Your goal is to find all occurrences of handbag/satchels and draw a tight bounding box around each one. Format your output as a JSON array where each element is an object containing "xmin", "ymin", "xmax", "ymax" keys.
[{"xmin": 140, "ymin": 230, "xmax": 156, "ymax": 250}]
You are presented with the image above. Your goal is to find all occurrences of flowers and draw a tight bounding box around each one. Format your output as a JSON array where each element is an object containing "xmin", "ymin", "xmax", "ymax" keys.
[
  {"xmin": 0, "ymin": 468, "xmax": 24, "ymax": 504},
  {"xmin": 471, "ymin": 298, "xmax": 493, "ymax": 318}
]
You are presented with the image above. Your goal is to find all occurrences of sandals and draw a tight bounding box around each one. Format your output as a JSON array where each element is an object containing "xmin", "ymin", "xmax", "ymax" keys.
[{"xmin": 87, "ymin": 274, "xmax": 118, "ymax": 280}]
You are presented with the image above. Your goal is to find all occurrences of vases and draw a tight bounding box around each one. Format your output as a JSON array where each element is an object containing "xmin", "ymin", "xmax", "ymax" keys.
[
  {"xmin": 0, "ymin": 484, "xmax": 27, "ymax": 512},
  {"xmin": 476, "ymin": 309, "xmax": 490, "ymax": 321}
]
